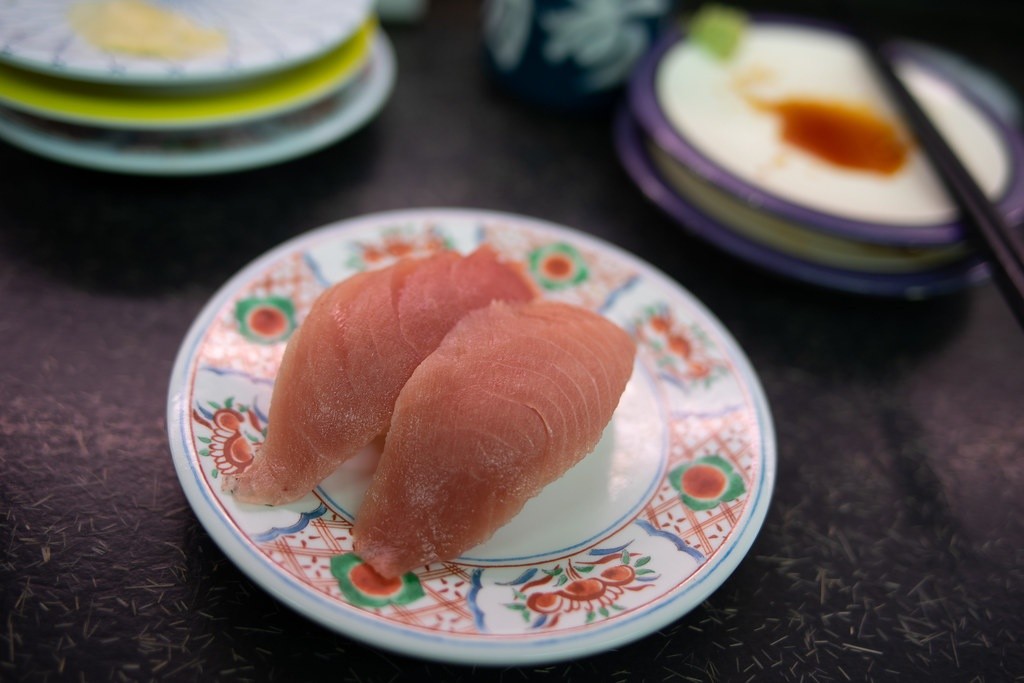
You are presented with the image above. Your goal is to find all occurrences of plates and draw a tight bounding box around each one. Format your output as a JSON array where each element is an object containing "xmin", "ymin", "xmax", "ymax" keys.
[
  {"xmin": 0, "ymin": 35, "xmax": 396, "ymax": 174},
  {"xmin": 165, "ymin": 208, "xmax": 777, "ymax": 662},
  {"xmin": 630, "ymin": 20, "xmax": 1024, "ymax": 249},
  {"xmin": 0, "ymin": 17, "xmax": 375, "ymax": 130},
  {"xmin": 0, "ymin": 0, "xmax": 373, "ymax": 84},
  {"xmin": 616, "ymin": 110, "xmax": 1024, "ymax": 294}
]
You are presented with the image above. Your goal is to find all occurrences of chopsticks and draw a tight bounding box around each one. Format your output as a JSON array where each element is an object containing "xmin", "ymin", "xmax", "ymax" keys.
[{"xmin": 857, "ymin": 35, "xmax": 1024, "ymax": 320}]
